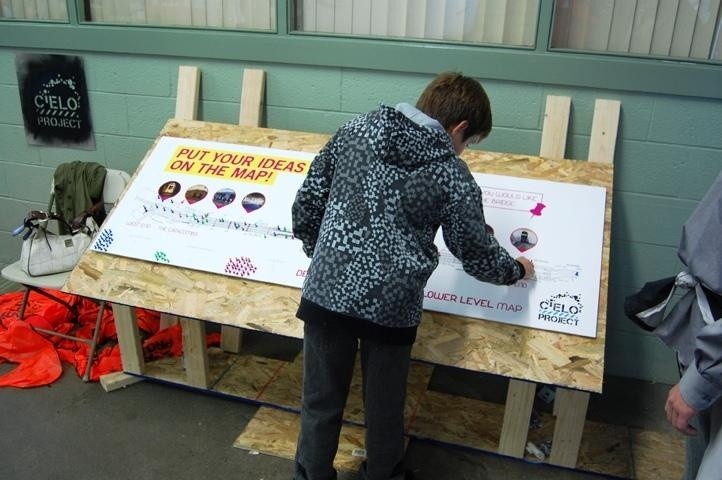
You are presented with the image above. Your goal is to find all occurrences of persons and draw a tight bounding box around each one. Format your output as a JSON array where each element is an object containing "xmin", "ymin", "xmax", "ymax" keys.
[
  {"xmin": 626, "ymin": 168, "xmax": 721, "ymax": 479},
  {"xmin": 293, "ymin": 72, "xmax": 533, "ymax": 479}
]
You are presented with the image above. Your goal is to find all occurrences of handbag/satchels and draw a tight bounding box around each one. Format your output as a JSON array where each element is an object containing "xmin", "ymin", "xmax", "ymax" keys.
[{"xmin": 19, "ymin": 216, "xmax": 100, "ymax": 278}]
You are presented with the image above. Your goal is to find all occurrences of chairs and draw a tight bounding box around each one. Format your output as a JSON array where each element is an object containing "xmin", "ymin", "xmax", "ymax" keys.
[{"xmin": 0, "ymin": 169, "xmax": 130, "ymax": 383}]
